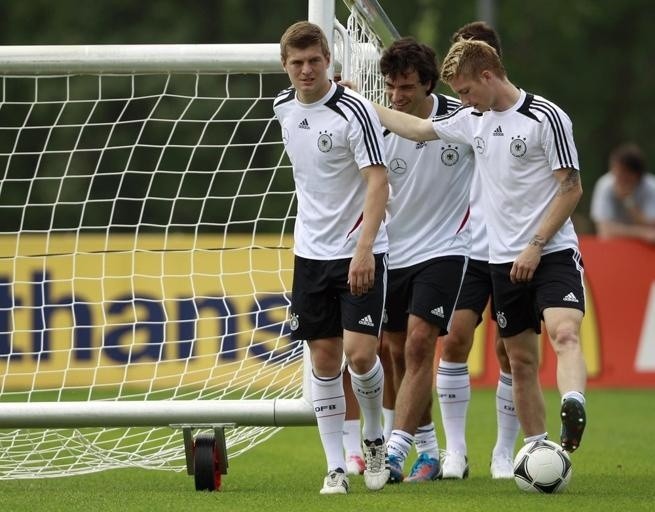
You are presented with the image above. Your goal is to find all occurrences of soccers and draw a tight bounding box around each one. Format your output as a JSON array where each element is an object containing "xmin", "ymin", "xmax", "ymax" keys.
[{"xmin": 514, "ymin": 440, "xmax": 573, "ymax": 494}]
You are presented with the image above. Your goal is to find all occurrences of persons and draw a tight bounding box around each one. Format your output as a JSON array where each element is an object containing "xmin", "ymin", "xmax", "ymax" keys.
[
  {"xmin": 339, "ymin": 330, "xmax": 396, "ymax": 475},
  {"xmin": 591, "ymin": 144, "xmax": 655, "ymax": 242},
  {"xmin": 438, "ymin": 23, "xmax": 518, "ymax": 480},
  {"xmin": 337, "ymin": 39, "xmax": 586, "ymax": 453},
  {"xmin": 272, "ymin": 21, "xmax": 390, "ymax": 496},
  {"xmin": 379, "ymin": 39, "xmax": 473, "ymax": 483}
]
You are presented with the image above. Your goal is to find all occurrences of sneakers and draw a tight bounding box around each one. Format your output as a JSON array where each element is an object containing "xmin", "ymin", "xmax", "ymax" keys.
[
  {"xmin": 490, "ymin": 450, "xmax": 514, "ymax": 480},
  {"xmin": 388, "ymin": 455, "xmax": 404, "ymax": 484},
  {"xmin": 402, "ymin": 453, "xmax": 441, "ymax": 482},
  {"xmin": 320, "ymin": 467, "xmax": 349, "ymax": 496},
  {"xmin": 345, "ymin": 455, "xmax": 365, "ymax": 476},
  {"xmin": 559, "ymin": 399, "xmax": 586, "ymax": 453},
  {"xmin": 440, "ymin": 448, "xmax": 469, "ymax": 480},
  {"xmin": 364, "ymin": 436, "xmax": 389, "ymax": 491}
]
